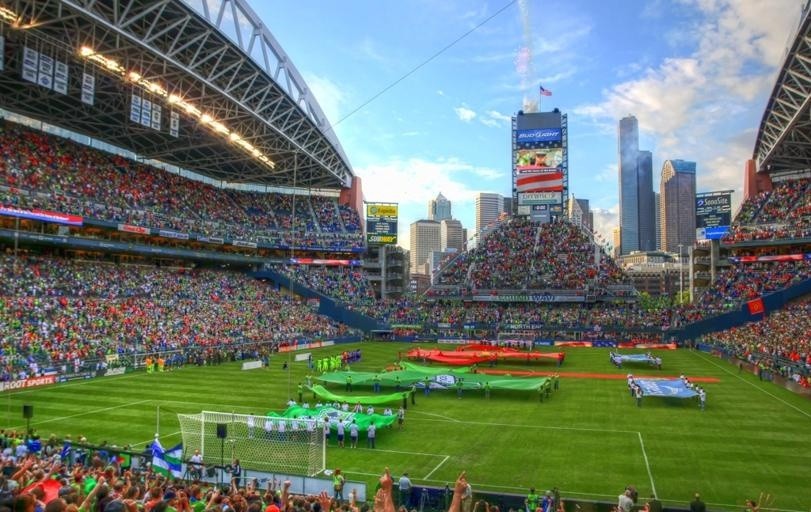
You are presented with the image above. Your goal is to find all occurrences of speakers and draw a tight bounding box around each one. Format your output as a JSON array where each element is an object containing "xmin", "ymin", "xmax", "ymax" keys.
[
  {"xmin": 23, "ymin": 406, "xmax": 33, "ymax": 418},
  {"xmin": 217, "ymin": 424, "xmax": 227, "ymax": 438}
]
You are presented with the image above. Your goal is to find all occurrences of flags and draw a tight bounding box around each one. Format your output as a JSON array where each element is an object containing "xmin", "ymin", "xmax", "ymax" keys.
[{"xmin": 541, "ymin": 87, "xmax": 553, "ymax": 97}]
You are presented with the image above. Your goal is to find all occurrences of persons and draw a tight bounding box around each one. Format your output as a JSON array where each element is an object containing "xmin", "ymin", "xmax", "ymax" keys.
[
  {"xmin": 444, "ymin": 469, "xmax": 770, "ymax": 512},
  {"xmin": 708, "ymin": 346, "xmax": 811, "ymax": 391},
  {"xmin": 0, "ymin": 427, "xmax": 414, "ymax": 511},
  {"xmin": 247, "ymin": 347, "xmax": 708, "ymax": 449},
  {"xmin": 0, "ymin": 347, "xmax": 247, "ymax": 380},
  {"xmin": 0, "ymin": 114, "xmax": 811, "ymax": 347}
]
